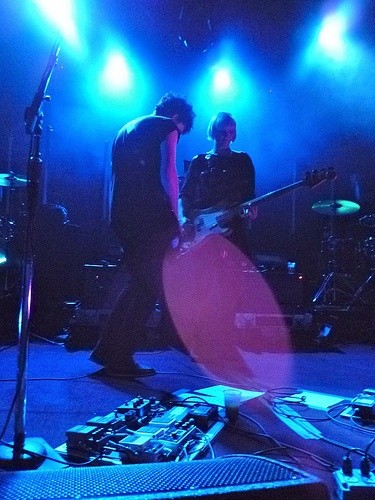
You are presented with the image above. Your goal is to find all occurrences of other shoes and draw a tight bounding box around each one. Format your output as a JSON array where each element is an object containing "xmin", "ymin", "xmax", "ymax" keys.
[
  {"xmin": 108, "ymin": 358, "xmax": 157, "ymax": 376},
  {"xmin": 89, "ymin": 349, "xmax": 106, "ymax": 366}
]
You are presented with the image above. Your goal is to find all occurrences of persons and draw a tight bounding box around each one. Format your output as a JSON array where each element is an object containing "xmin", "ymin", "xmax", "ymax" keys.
[
  {"xmin": 89, "ymin": 94, "xmax": 195, "ymax": 379},
  {"xmin": 180, "ymin": 113, "xmax": 258, "ymax": 362}
]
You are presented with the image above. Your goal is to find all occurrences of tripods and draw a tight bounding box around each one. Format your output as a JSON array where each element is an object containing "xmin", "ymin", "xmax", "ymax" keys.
[{"xmin": 311, "ymin": 179, "xmax": 357, "ymax": 304}]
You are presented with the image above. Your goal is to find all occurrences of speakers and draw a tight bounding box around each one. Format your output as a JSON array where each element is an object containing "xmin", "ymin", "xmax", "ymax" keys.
[
  {"xmin": 262, "ymin": 271, "xmax": 306, "ymax": 307},
  {"xmin": 81, "ymin": 264, "xmax": 121, "ymax": 308}
]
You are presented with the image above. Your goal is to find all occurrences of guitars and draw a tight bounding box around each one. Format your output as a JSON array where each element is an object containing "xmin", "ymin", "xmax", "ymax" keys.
[{"xmin": 190, "ymin": 167, "xmax": 337, "ymax": 252}]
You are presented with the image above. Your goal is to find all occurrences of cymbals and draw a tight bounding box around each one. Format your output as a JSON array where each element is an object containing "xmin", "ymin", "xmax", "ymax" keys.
[
  {"xmin": 312, "ymin": 200, "xmax": 361, "ymax": 216},
  {"xmin": 359, "ymin": 214, "xmax": 375, "ymax": 226},
  {"xmin": 0, "ymin": 172, "xmax": 41, "ymax": 187}
]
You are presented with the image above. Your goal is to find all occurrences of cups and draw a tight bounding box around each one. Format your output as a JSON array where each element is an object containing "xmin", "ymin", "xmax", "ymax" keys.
[{"xmin": 287, "ymin": 262, "xmax": 296, "ymax": 274}]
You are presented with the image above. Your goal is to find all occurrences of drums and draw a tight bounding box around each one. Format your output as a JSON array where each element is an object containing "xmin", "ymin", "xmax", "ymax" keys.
[
  {"xmin": 361, "ymin": 237, "xmax": 375, "ymax": 271},
  {"xmin": 325, "ymin": 238, "xmax": 359, "ymax": 276}
]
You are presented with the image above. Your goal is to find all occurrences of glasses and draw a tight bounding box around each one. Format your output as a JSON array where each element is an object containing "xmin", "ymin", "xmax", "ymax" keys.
[{"xmin": 219, "ymin": 130, "xmax": 234, "ymax": 136}]
